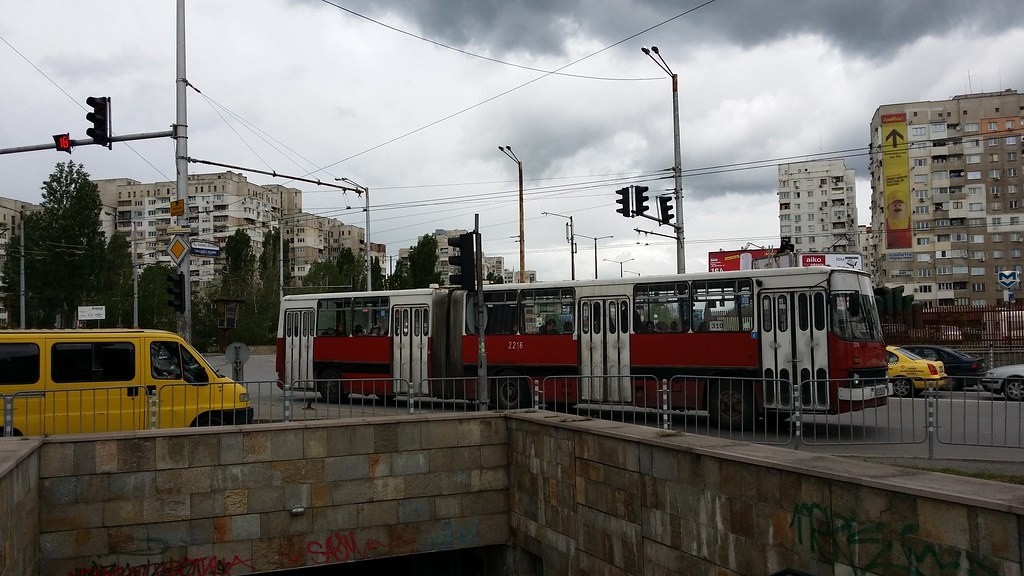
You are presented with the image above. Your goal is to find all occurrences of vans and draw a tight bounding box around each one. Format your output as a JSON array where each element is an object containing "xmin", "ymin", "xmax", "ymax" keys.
[
  {"xmin": 0, "ymin": 328, "xmax": 254, "ymax": 437},
  {"xmin": 982, "ymin": 311, "xmax": 1024, "ymax": 346}
]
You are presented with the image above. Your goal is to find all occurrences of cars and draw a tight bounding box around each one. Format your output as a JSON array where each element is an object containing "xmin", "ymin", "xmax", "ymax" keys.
[
  {"xmin": 932, "ymin": 326, "xmax": 962, "ymax": 341},
  {"xmin": 982, "ymin": 364, "xmax": 1024, "ymax": 402},
  {"xmin": 886, "ymin": 346, "xmax": 948, "ymax": 398},
  {"xmin": 899, "ymin": 345, "xmax": 987, "ymax": 391}
]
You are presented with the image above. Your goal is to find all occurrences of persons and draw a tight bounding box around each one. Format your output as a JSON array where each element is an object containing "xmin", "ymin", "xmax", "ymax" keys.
[
  {"xmin": 152, "ymin": 367, "xmax": 175, "ymax": 381},
  {"xmin": 512, "ymin": 300, "xmax": 709, "ymax": 335},
  {"xmin": 322, "ymin": 322, "xmax": 399, "ymax": 337},
  {"xmin": 774, "ymin": 256, "xmax": 782, "ymax": 268}
]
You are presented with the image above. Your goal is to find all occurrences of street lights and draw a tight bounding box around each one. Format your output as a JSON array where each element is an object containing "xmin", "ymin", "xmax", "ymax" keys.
[
  {"xmin": 104, "ymin": 212, "xmax": 139, "ymax": 327},
  {"xmin": 602, "ymin": 258, "xmax": 634, "ymax": 278},
  {"xmin": 542, "ymin": 210, "xmax": 575, "ymax": 281},
  {"xmin": 641, "ymin": 45, "xmax": 686, "ymax": 274},
  {"xmin": 498, "ymin": 144, "xmax": 525, "ymax": 282},
  {"xmin": 337, "ymin": 176, "xmax": 371, "ymax": 292},
  {"xmin": 573, "ymin": 233, "xmax": 614, "ymax": 279}
]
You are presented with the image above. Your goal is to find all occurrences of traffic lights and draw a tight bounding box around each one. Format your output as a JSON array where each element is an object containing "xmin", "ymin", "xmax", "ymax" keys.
[
  {"xmin": 86, "ymin": 96, "xmax": 109, "ymax": 145},
  {"xmin": 447, "ymin": 233, "xmax": 475, "ymax": 290},
  {"xmin": 656, "ymin": 196, "xmax": 674, "ymax": 226},
  {"xmin": 615, "ymin": 187, "xmax": 632, "ymax": 217},
  {"xmin": 633, "ymin": 186, "xmax": 649, "ymax": 216},
  {"xmin": 52, "ymin": 134, "xmax": 69, "ymax": 152}
]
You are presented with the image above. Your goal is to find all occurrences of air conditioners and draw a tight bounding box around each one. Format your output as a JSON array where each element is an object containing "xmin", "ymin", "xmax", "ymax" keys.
[{"xmin": 980, "ymin": 257, "xmax": 985, "ymax": 261}]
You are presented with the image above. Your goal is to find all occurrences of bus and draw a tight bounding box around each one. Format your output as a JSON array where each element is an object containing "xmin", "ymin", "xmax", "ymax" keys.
[{"xmin": 273, "ymin": 264, "xmax": 895, "ymax": 427}]
